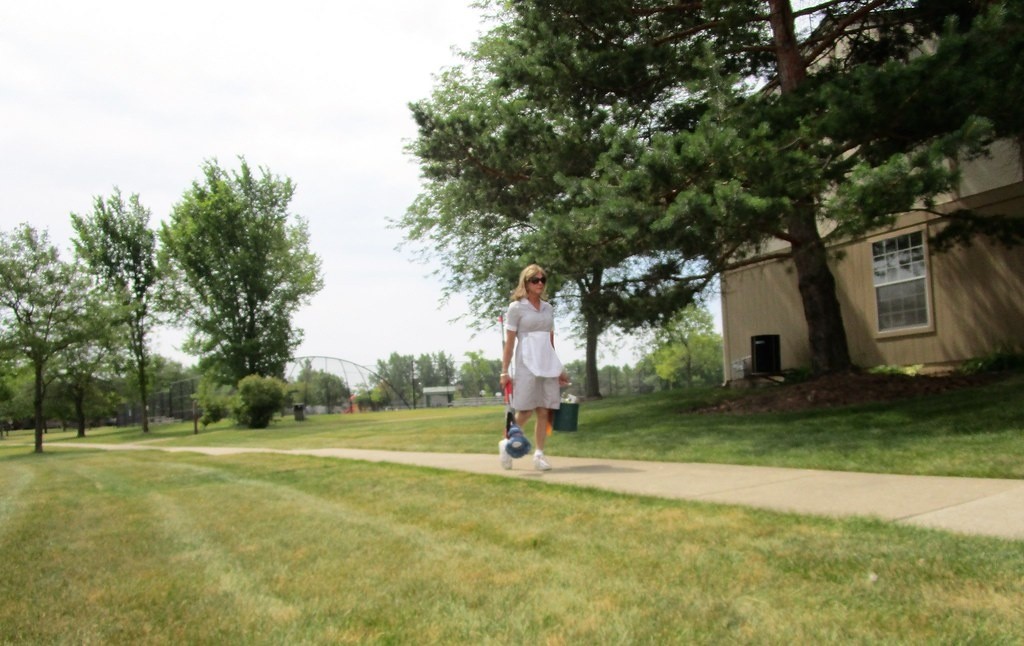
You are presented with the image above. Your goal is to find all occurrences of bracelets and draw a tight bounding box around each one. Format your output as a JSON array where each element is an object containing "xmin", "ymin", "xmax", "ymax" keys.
[{"xmin": 500, "ymin": 373, "xmax": 509, "ymax": 376}]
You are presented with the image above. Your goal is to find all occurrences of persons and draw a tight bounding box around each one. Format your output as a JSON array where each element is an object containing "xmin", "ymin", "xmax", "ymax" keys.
[{"xmin": 499, "ymin": 264, "xmax": 569, "ymax": 470}]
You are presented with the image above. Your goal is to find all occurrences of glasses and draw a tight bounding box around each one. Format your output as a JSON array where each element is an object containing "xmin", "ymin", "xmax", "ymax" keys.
[{"xmin": 528, "ymin": 276, "xmax": 546, "ymax": 284}]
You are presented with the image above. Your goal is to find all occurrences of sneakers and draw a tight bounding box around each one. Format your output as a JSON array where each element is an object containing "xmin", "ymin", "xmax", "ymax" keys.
[
  {"xmin": 533, "ymin": 456, "xmax": 551, "ymax": 470},
  {"xmin": 498, "ymin": 441, "xmax": 512, "ymax": 469}
]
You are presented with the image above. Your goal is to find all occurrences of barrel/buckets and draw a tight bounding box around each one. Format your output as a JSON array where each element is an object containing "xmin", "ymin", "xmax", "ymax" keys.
[{"xmin": 553, "ymin": 402, "xmax": 579, "ymax": 432}]
